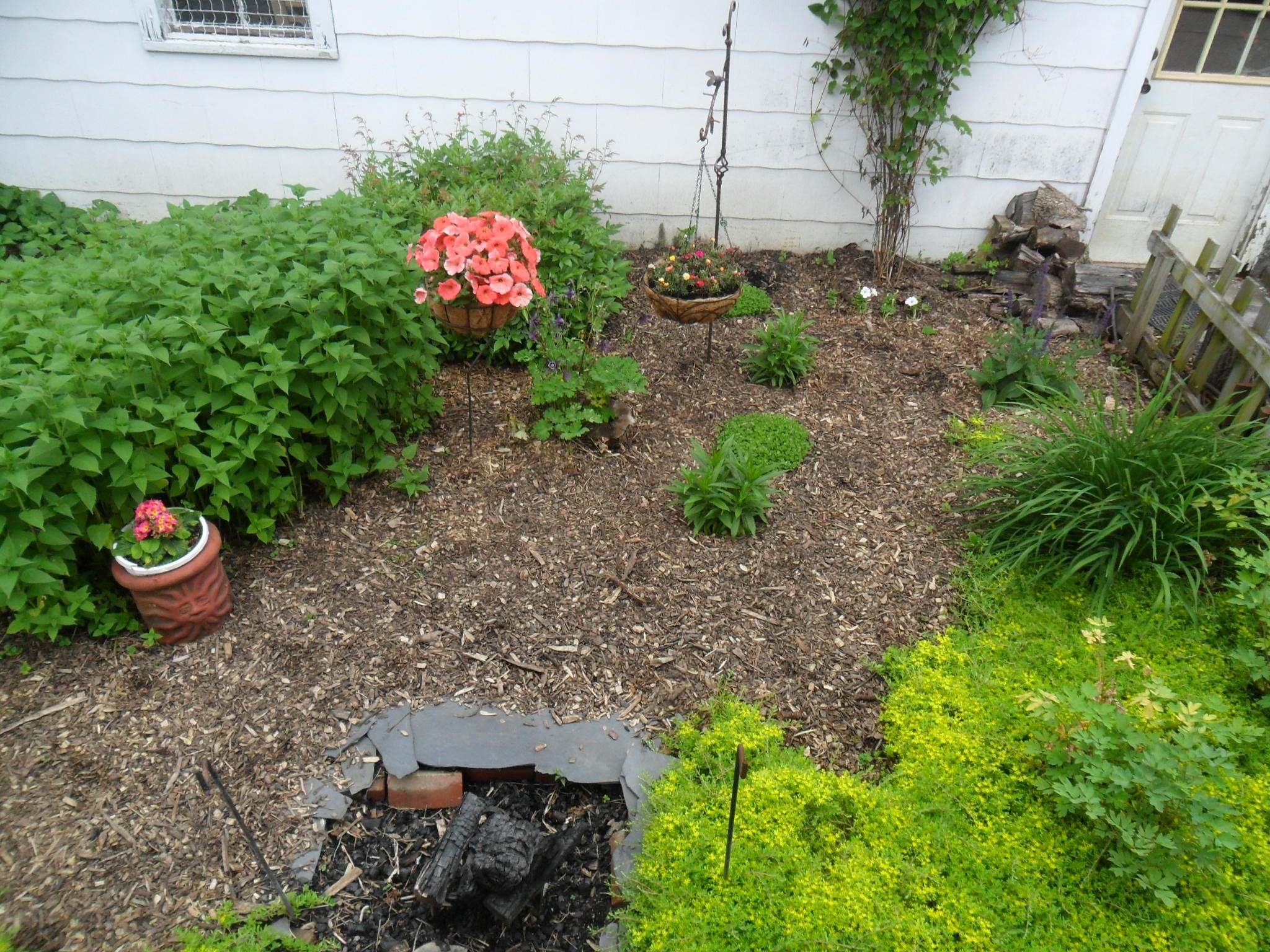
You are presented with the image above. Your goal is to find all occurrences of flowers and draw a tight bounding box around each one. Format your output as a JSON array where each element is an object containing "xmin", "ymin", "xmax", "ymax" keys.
[
  {"xmin": 406, "ymin": 210, "xmax": 547, "ymax": 308},
  {"xmin": 110, "ymin": 498, "xmax": 203, "ymax": 570},
  {"xmin": 647, "ymin": 233, "xmax": 746, "ymax": 294}
]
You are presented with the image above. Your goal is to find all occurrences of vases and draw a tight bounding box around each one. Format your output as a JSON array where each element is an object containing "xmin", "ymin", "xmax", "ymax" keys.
[
  {"xmin": 111, "ymin": 507, "xmax": 235, "ymax": 647},
  {"xmin": 643, "ymin": 272, "xmax": 743, "ymax": 324},
  {"xmin": 425, "ymin": 280, "xmax": 520, "ymax": 337}
]
